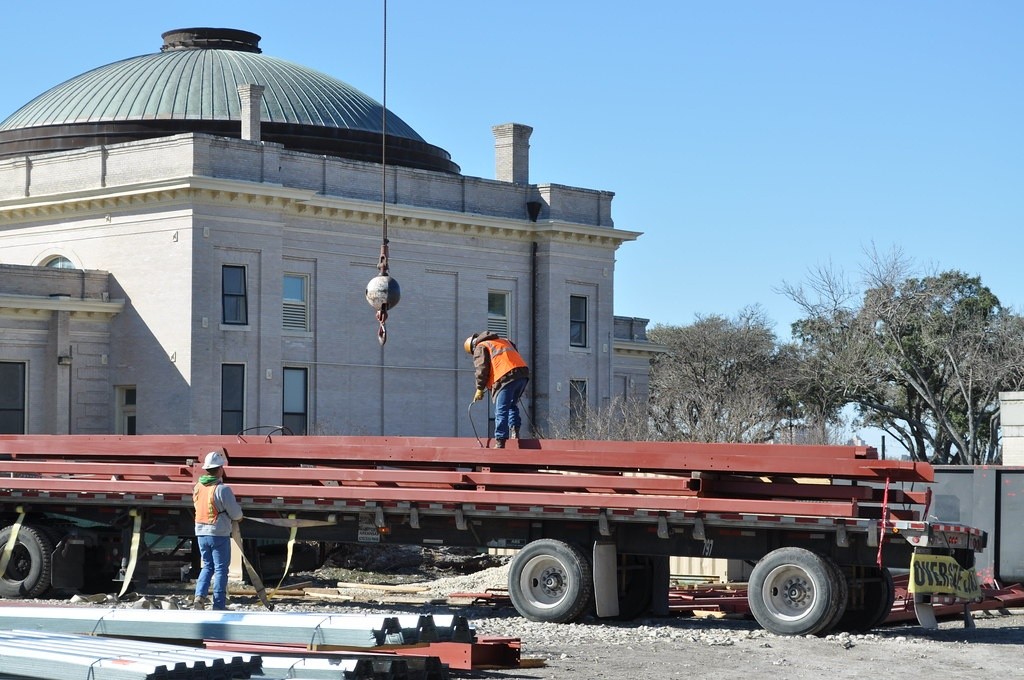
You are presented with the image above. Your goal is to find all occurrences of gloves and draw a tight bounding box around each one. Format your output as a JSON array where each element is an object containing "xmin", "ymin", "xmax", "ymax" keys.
[{"xmin": 474, "ymin": 389, "xmax": 482, "ymax": 402}]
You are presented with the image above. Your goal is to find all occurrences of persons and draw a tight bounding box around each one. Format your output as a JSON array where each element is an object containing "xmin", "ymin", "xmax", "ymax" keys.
[
  {"xmin": 192, "ymin": 452, "xmax": 243, "ymax": 611},
  {"xmin": 464, "ymin": 331, "xmax": 530, "ymax": 448}
]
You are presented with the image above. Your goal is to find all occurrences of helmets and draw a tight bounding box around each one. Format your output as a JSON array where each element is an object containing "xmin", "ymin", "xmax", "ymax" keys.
[
  {"xmin": 202, "ymin": 452, "xmax": 223, "ymax": 469},
  {"xmin": 464, "ymin": 334, "xmax": 478, "ymax": 355}
]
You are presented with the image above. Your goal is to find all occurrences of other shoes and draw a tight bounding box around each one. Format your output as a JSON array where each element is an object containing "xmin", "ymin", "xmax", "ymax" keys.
[
  {"xmin": 194, "ymin": 596, "xmax": 204, "ymax": 610},
  {"xmin": 213, "ymin": 605, "xmax": 228, "ymax": 610}
]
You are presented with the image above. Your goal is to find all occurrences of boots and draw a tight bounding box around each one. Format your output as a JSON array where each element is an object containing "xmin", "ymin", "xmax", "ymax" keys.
[
  {"xmin": 495, "ymin": 439, "xmax": 505, "ymax": 448},
  {"xmin": 511, "ymin": 426, "xmax": 518, "ymax": 439}
]
